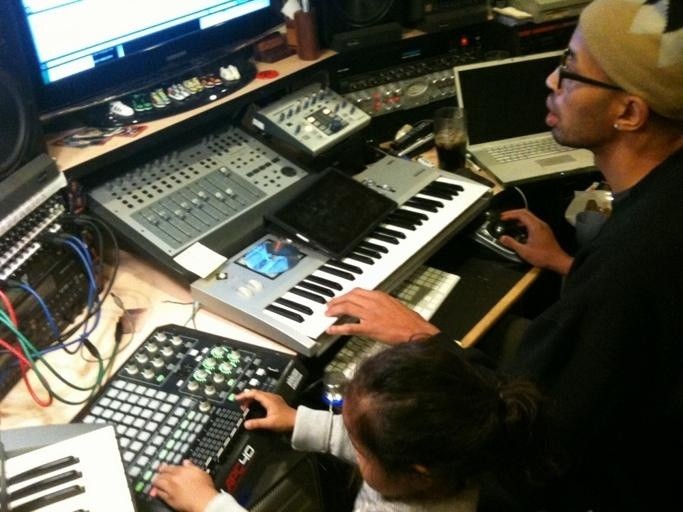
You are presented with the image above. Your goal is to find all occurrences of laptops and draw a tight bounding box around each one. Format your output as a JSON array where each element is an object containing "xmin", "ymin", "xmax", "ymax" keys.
[{"xmin": 453, "ymin": 49, "xmax": 600, "ymax": 188}]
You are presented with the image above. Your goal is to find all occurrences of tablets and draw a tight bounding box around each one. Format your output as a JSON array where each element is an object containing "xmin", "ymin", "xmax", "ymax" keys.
[{"xmin": 266, "ymin": 165, "xmax": 399, "ymax": 257}]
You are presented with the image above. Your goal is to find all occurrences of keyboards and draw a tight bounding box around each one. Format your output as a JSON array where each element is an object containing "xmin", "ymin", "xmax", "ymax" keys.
[{"xmin": 324, "ymin": 264, "xmax": 461, "ymax": 380}]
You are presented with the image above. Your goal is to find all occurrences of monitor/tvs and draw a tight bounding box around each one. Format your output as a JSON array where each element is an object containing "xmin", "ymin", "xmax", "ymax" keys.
[{"xmin": 1, "ymin": 0, "xmax": 286, "ymax": 126}]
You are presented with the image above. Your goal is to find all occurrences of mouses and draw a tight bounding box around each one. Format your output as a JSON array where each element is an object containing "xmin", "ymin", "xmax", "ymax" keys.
[{"xmin": 486, "ymin": 218, "xmax": 527, "ymax": 246}]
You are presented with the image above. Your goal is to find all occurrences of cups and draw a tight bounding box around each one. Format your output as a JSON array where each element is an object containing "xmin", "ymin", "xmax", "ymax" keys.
[{"xmin": 432, "ymin": 106, "xmax": 469, "ymax": 171}]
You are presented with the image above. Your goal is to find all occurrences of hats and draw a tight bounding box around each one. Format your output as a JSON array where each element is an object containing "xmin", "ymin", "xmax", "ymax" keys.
[{"xmin": 581, "ymin": 0, "xmax": 683, "ymax": 120}]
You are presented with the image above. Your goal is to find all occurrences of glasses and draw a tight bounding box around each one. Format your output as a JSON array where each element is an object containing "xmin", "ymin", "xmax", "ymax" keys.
[{"xmin": 555, "ymin": 45, "xmax": 625, "ymax": 97}]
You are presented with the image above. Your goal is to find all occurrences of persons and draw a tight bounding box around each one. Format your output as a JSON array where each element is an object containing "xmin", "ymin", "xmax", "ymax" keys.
[
  {"xmin": 147, "ymin": 331, "xmax": 502, "ymax": 512},
  {"xmin": 319, "ymin": 1, "xmax": 682, "ymax": 512}
]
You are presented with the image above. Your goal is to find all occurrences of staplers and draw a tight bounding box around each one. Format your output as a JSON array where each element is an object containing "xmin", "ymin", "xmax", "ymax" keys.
[{"xmin": 389, "ymin": 120, "xmax": 434, "ymax": 159}]
